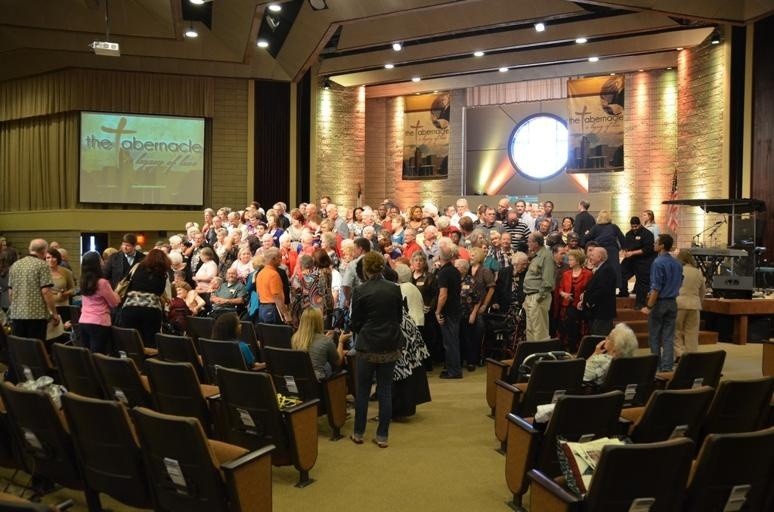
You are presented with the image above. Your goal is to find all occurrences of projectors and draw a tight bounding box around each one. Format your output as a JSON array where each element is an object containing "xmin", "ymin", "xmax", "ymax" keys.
[{"xmin": 88, "ymin": 39, "xmax": 123, "ymax": 58}]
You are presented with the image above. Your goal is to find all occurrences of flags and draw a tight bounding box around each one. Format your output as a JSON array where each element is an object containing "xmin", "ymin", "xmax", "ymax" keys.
[{"xmin": 664, "ymin": 168, "xmax": 680, "ymax": 236}]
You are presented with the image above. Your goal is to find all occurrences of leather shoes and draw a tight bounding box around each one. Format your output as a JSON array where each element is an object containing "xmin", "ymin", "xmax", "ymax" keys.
[
  {"xmin": 439, "ymin": 374, "xmax": 463, "ymax": 379},
  {"xmin": 441, "ymin": 371, "xmax": 448, "ymax": 374}
]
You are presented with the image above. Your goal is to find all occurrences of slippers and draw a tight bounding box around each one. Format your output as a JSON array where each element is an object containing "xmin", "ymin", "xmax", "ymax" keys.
[
  {"xmin": 372, "ymin": 437, "xmax": 388, "ymax": 448},
  {"xmin": 350, "ymin": 435, "xmax": 364, "ymax": 445}
]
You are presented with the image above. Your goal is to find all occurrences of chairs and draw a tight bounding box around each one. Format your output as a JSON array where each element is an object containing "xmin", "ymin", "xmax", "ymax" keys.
[
  {"xmin": 212, "ymin": 365, "xmax": 320, "ymax": 488},
  {"xmin": 50, "ymin": 342, "xmax": 105, "ymax": 399},
  {"xmin": 688, "ymin": 426, "xmax": 774, "ymax": 512},
  {"xmin": 111, "ymin": 325, "xmax": 158, "ymax": 372},
  {"xmin": 155, "ymin": 333, "xmax": 206, "ymax": 382},
  {"xmin": 263, "ymin": 346, "xmax": 348, "ymax": 442},
  {"xmin": 493, "ymin": 358, "xmax": 586, "ymax": 457},
  {"xmin": 323, "ymin": 329, "xmax": 357, "ymax": 398},
  {"xmin": 485, "ymin": 337, "xmax": 561, "ymax": 422},
  {"xmin": 0, "ymin": 411, "xmax": 36, "ymax": 498},
  {"xmin": 256, "ymin": 322, "xmax": 294, "ymax": 370},
  {"xmin": 697, "ymin": 375, "xmax": 774, "ymax": 435},
  {"xmin": 0, "ymin": 381, "xmax": 101, "ymax": 512},
  {"xmin": 504, "ymin": 390, "xmax": 624, "ymax": 512},
  {"xmin": 7, "ymin": 334, "xmax": 59, "ymax": 385},
  {"xmin": 198, "ymin": 338, "xmax": 266, "ymax": 385},
  {"xmin": 585, "ymin": 353, "xmax": 659, "ymax": 408},
  {"xmin": 186, "ymin": 316, "xmax": 215, "ymax": 355},
  {"xmin": 0, "ymin": 492, "xmax": 73, "ymax": 512},
  {"xmin": 92, "ymin": 352, "xmax": 154, "ymax": 411},
  {"xmin": 143, "ymin": 358, "xmax": 221, "ymax": 441},
  {"xmin": 619, "ymin": 386, "xmax": 714, "ymax": 444},
  {"xmin": 239, "ymin": 320, "xmax": 259, "ymax": 355},
  {"xmin": 653, "ymin": 349, "xmax": 727, "ymax": 390},
  {"xmin": 563, "ymin": 334, "xmax": 608, "ymax": 359},
  {"xmin": 131, "ymin": 406, "xmax": 276, "ymax": 512},
  {"xmin": 56, "ymin": 304, "xmax": 81, "ymax": 323},
  {"xmin": 60, "ymin": 391, "xmax": 156, "ymax": 512},
  {"xmin": 528, "ymin": 437, "xmax": 695, "ymax": 512}
]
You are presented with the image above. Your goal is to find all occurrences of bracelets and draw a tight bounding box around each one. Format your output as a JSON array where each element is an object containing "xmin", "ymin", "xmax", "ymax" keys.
[{"xmin": 646, "ymin": 304, "xmax": 656, "ymax": 309}]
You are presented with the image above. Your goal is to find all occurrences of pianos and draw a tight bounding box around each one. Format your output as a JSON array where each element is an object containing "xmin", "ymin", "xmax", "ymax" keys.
[{"xmin": 689, "ymin": 245, "xmax": 749, "ymax": 257}]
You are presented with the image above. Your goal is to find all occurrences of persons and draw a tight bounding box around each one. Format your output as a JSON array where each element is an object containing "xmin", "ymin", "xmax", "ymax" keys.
[
  {"xmin": 435, "ymin": 95, "xmax": 449, "ymax": 129},
  {"xmin": 641, "ymin": 234, "xmax": 684, "ymax": 373},
  {"xmin": 606, "ymin": 77, "xmax": 625, "ymax": 114},
  {"xmin": 672, "ymin": 247, "xmax": 706, "ymax": 364},
  {"xmin": 583, "ymin": 323, "xmax": 638, "ymax": 386},
  {"xmin": 470, "ymin": 192, "xmax": 625, "ymax": 342},
  {"xmin": 616, "ymin": 216, "xmax": 654, "ymax": 311},
  {"xmin": 641, "ymin": 210, "xmax": 659, "ymax": 241}
]
